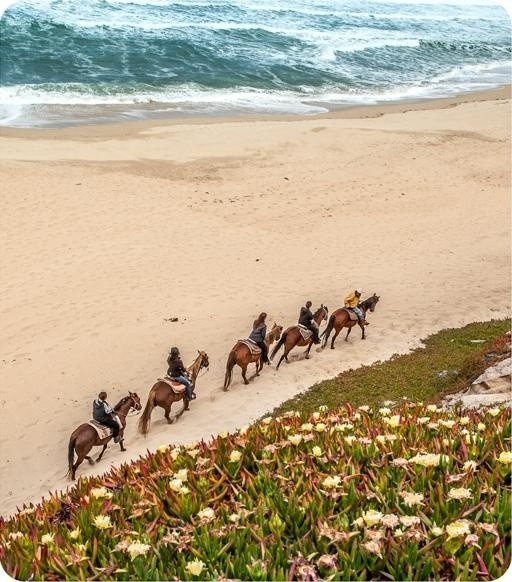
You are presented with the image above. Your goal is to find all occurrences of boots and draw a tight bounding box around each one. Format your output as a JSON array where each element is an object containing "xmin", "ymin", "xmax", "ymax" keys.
[{"xmin": 360, "ymin": 319, "xmax": 370, "ymax": 327}]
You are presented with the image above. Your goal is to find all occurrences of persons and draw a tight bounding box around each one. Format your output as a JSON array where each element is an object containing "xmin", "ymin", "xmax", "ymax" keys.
[
  {"xmin": 93, "ymin": 392, "xmax": 123, "ymax": 444},
  {"xmin": 299, "ymin": 301, "xmax": 321, "ymax": 344},
  {"xmin": 345, "ymin": 287, "xmax": 369, "ymax": 325},
  {"xmin": 168, "ymin": 347, "xmax": 194, "ymax": 401},
  {"xmin": 248, "ymin": 312, "xmax": 270, "ymax": 364}
]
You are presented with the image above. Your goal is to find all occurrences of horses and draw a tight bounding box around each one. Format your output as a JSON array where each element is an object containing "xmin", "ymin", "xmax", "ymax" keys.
[
  {"xmin": 65, "ymin": 390, "xmax": 143, "ymax": 482},
  {"xmin": 270, "ymin": 303, "xmax": 329, "ymax": 371},
  {"xmin": 223, "ymin": 322, "xmax": 283, "ymax": 392},
  {"xmin": 319, "ymin": 292, "xmax": 381, "ymax": 350},
  {"xmin": 137, "ymin": 349, "xmax": 209, "ymax": 439}
]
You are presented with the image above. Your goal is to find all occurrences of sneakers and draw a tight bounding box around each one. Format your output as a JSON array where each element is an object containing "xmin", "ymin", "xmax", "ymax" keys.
[{"xmin": 114, "ymin": 434, "xmax": 125, "ymax": 443}]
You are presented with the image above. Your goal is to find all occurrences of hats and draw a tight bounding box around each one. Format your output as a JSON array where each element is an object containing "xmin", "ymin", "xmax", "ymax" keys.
[
  {"xmin": 169, "ymin": 347, "xmax": 180, "ymax": 354},
  {"xmin": 357, "ymin": 287, "xmax": 363, "ymax": 293}
]
